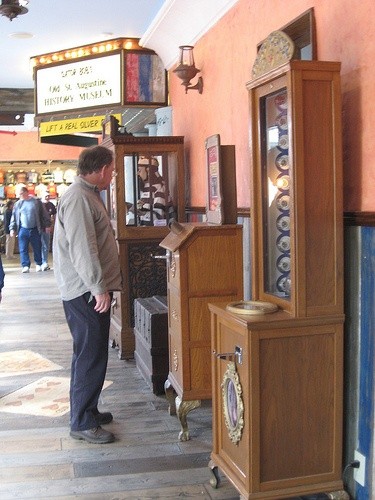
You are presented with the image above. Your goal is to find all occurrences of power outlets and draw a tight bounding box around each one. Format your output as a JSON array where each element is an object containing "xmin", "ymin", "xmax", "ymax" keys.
[{"xmin": 352, "ymin": 449, "xmax": 365, "ymax": 488}]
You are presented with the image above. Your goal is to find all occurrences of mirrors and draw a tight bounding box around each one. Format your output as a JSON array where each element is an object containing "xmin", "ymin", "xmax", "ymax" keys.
[{"xmin": 262, "ymin": 87, "xmax": 292, "ymax": 300}]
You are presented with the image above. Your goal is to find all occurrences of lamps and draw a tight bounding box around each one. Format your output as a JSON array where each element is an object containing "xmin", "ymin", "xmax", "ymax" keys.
[
  {"xmin": 0, "ymin": 0, "xmax": 30, "ymax": 20},
  {"xmin": 172, "ymin": 46, "xmax": 204, "ymax": 95}
]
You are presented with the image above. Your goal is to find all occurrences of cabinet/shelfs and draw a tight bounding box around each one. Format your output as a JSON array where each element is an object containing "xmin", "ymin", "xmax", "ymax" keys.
[
  {"xmin": 210, "ymin": 57, "xmax": 348, "ymax": 500},
  {"xmin": 100, "ymin": 114, "xmax": 185, "ymax": 359},
  {"xmin": 134, "ymin": 295, "xmax": 171, "ymax": 396},
  {"xmin": 159, "ymin": 221, "xmax": 245, "ymax": 443}
]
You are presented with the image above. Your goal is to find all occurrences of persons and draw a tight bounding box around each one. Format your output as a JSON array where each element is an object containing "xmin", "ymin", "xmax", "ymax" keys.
[
  {"xmin": 53, "ymin": 146, "xmax": 123, "ymax": 445},
  {"xmin": 4, "ymin": 201, "xmax": 17, "ymax": 260},
  {"xmin": 41, "ymin": 192, "xmax": 57, "ymax": 254},
  {"xmin": 124, "ymin": 156, "xmax": 173, "ymax": 226},
  {"xmin": 9, "ymin": 186, "xmax": 51, "ymax": 272},
  {"xmin": 0, "ymin": 168, "xmax": 75, "ymax": 221}
]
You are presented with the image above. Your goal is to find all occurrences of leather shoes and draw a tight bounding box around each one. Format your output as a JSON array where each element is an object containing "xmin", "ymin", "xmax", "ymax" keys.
[
  {"xmin": 95, "ymin": 410, "xmax": 113, "ymax": 425},
  {"xmin": 70, "ymin": 425, "xmax": 116, "ymax": 444}
]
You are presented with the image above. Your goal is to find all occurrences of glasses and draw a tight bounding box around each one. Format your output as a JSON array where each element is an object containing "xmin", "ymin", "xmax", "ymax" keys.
[{"xmin": 108, "ymin": 166, "xmax": 119, "ymax": 177}]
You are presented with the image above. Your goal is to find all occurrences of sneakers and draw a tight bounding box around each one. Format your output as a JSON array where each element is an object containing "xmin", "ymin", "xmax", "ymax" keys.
[
  {"xmin": 22, "ymin": 265, "xmax": 30, "ymax": 273},
  {"xmin": 41, "ymin": 263, "xmax": 50, "ymax": 271},
  {"xmin": 36, "ymin": 265, "xmax": 42, "ymax": 272}
]
